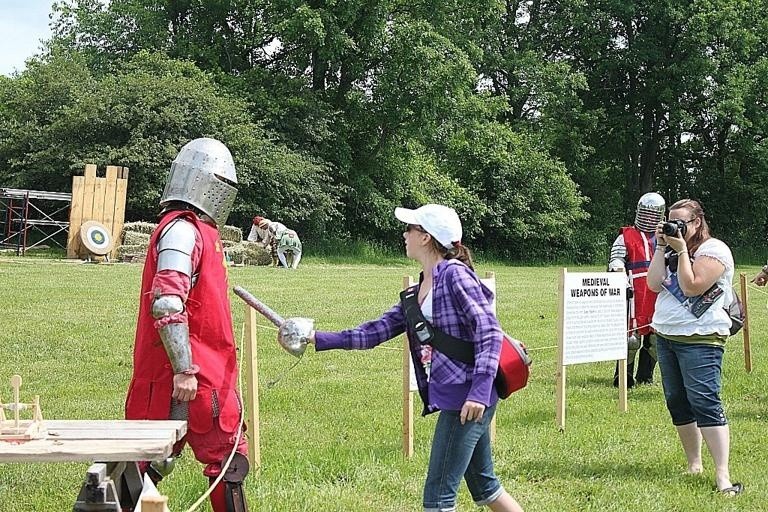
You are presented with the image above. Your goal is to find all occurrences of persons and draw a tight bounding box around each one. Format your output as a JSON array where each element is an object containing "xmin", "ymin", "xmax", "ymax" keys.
[
  {"xmin": 247, "ymin": 214, "xmax": 266, "ymax": 242},
  {"xmin": 275, "ymin": 230, "xmax": 302, "ymax": 271},
  {"xmin": 122, "ymin": 138, "xmax": 251, "ymax": 512},
  {"xmin": 606, "ymin": 192, "xmax": 669, "ymax": 391},
  {"xmin": 646, "ymin": 197, "xmax": 745, "ymax": 497},
  {"xmin": 258, "ymin": 218, "xmax": 290, "ymax": 267},
  {"xmin": 298, "ymin": 204, "xmax": 525, "ymax": 512}
]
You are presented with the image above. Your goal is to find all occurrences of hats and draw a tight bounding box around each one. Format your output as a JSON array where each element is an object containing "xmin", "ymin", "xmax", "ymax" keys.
[
  {"xmin": 394, "ymin": 203, "xmax": 463, "ymax": 249},
  {"xmin": 254, "ymin": 217, "xmax": 268, "ymax": 228}
]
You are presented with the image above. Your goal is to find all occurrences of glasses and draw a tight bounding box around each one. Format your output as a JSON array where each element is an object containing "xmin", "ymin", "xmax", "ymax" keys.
[{"xmin": 403, "ymin": 222, "xmax": 425, "ymax": 232}]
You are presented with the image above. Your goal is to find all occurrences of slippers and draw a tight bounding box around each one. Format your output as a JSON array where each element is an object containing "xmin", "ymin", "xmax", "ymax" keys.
[{"xmin": 716, "ymin": 483, "xmax": 743, "ymax": 499}]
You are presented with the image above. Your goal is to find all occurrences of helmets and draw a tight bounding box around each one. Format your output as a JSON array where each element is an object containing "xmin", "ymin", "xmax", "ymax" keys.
[
  {"xmin": 636, "ymin": 193, "xmax": 666, "ymax": 233},
  {"xmin": 158, "ymin": 137, "xmax": 239, "ymax": 230}
]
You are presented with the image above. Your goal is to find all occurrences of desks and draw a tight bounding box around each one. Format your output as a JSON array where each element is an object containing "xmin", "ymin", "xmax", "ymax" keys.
[{"xmin": 0, "ymin": 416, "xmax": 191, "ymax": 510}]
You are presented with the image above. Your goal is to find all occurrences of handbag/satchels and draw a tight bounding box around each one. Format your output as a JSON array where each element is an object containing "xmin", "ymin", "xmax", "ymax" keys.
[
  {"xmin": 496, "ymin": 331, "xmax": 532, "ymax": 400},
  {"xmin": 728, "ymin": 287, "xmax": 745, "ymax": 336}
]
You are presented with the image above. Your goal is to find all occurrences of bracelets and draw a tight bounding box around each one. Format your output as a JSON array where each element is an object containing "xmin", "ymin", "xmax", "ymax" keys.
[
  {"xmin": 677, "ymin": 249, "xmax": 688, "ymax": 256},
  {"xmin": 656, "ymin": 247, "xmax": 667, "ymax": 251}
]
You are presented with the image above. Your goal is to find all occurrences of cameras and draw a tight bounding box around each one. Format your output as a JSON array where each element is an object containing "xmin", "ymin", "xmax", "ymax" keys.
[{"xmin": 662, "ymin": 219, "xmax": 687, "ymax": 238}]
